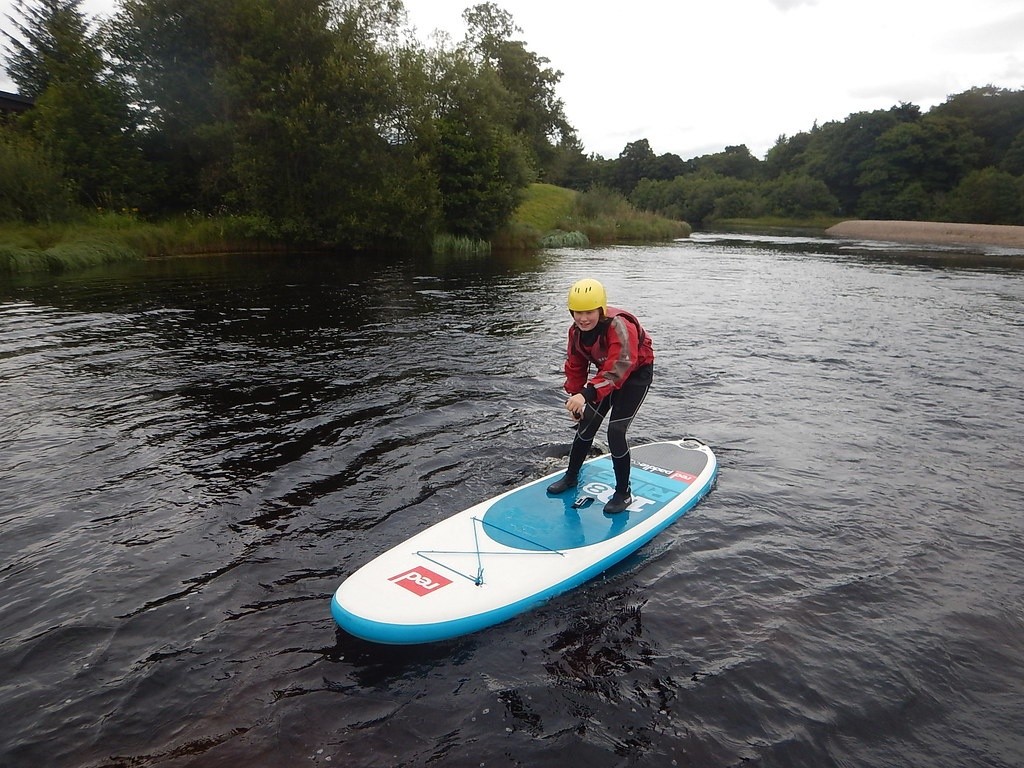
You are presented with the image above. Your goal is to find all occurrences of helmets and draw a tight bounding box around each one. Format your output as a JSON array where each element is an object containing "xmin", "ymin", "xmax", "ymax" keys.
[{"xmin": 568, "ymin": 279, "xmax": 606, "ymax": 320}]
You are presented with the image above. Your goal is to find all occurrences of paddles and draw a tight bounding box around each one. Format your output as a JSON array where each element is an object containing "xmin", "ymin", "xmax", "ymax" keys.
[{"xmin": 564, "ymin": 400, "xmax": 583, "ymax": 428}]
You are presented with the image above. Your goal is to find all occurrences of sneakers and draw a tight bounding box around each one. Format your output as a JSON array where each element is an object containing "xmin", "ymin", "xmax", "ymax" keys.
[
  {"xmin": 603, "ymin": 491, "xmax": 632, "ymax": 513},
  {"xmin": 547, "ymin": 470, "xmax": 578, "ymax": 494}
]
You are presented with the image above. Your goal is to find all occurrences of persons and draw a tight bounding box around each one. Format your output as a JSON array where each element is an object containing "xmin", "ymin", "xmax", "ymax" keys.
[{"xmin": 546, "ymin": 278, "xmax": 654, "ymax": 514}]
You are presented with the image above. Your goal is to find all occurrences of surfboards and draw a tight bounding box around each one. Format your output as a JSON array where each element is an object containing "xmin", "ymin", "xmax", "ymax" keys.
[{"xmin": 327, "ymin": 432, "xmax": 718, "ymax": 650}]
